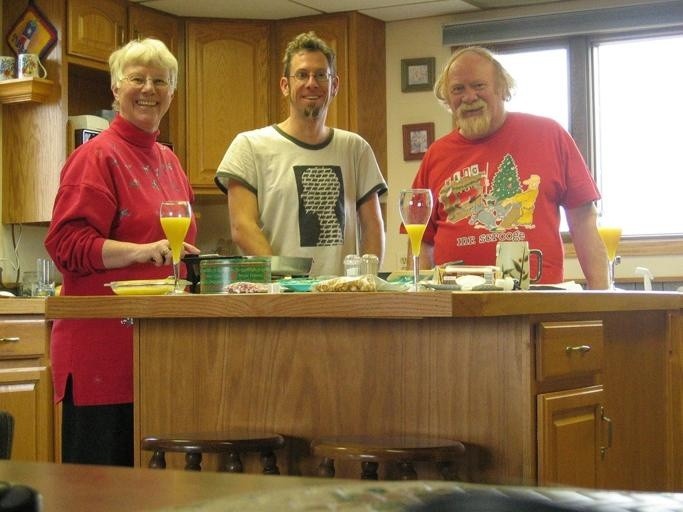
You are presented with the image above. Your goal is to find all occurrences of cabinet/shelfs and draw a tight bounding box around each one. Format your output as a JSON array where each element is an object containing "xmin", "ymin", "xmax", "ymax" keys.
[
  {"xmin": 0, "ymin": 0, "xmax": 386, "ymax": 227},
  {"xmin": 0, "ymin": 298, "xmax": 63, "ymax": 462},
  {"xmin": 530, "ymin": 311, "xmax": 613, "ymax": 493}
]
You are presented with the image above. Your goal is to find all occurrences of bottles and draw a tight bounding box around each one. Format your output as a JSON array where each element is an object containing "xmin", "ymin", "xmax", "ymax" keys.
[{"xmin": 342, "ymin": 253, "xmax": 379, "ymax": 278}]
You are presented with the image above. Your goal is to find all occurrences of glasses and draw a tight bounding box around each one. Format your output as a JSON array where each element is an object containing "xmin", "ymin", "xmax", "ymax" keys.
[
  {"xmin": 285, "ymin": 70, "xmax": 334, "ymax": 81},
  {"xmin": 119, "ymin": 73, "xmax": 169, "ymax": 89}
]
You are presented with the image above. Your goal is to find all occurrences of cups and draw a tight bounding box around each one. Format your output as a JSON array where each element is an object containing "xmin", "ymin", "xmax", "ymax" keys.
[
  {"xmin": 0, "ymin": 54, "xmax": 49, "ymax": 84},
  {"xmin": 495, "ymin": 241, "xmax": 542, "ymax": 292}
]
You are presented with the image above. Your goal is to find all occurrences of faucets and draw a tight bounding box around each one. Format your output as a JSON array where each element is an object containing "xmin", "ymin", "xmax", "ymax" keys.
[{"xmin": 608, "ymin": 256, "xmax": 620, "ymax": 290}]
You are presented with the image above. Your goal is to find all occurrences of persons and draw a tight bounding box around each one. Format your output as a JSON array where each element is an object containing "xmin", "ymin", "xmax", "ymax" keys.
[
  {"xmin": 403, "ymin": 46, "xmax": 614, "ymax": 291},
  {"xmin": 41, "ymin": 38, "xmax": 198, "ymax": 466},
  {"xmin": 214, "ymin": 31, "xmax": 390, "ymax": 279}
]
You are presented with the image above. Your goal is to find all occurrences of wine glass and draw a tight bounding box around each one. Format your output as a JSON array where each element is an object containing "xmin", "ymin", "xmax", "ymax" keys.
[
  {"xmin": 158, "ymin": 201, "xmax": 191, "ymax": 295},
  {"xmin": 597, "ymin": 209, "xmax": 624, "ymax": 292},
  {"xmin": 401, "ymin": 189, "xmax": 433, "ymax": 294}
]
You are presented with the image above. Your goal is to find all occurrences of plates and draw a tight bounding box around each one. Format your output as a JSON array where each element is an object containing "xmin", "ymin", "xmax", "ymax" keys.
[
  {"xmin": 421, "ymin": 284, "xmax": 502, "ymax": 294},
  {"xmin": 104, "ymin": 279, "xmax": 191, "ymax": 296},
  {"xmin": 278, "ymin": 278, "xmax": 320, "ymax": 291}
]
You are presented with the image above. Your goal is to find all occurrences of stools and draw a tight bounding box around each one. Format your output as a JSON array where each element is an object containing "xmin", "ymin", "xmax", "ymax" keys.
[
  {"xmin": 310, "ymin": 438, "xmax": 467, "ymax": 480},
  {"xmin": 141, "ymin": 432, "xmax": 285, "ymax": 474}
]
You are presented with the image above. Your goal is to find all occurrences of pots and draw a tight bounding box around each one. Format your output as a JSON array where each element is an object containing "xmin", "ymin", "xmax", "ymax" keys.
[{"xmin": 181, "ymin": 255, "xmax": 313, "ymax": 283}]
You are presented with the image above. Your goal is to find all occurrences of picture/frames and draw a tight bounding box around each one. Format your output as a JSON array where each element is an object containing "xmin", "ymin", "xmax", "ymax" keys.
[
  {"xmin": 400, "ymin": 56, "xmax": 436, "ymax": 92},
  {"xmin": 402, "ymin": 122, "xmax": 435, "ymax": 162}
]
